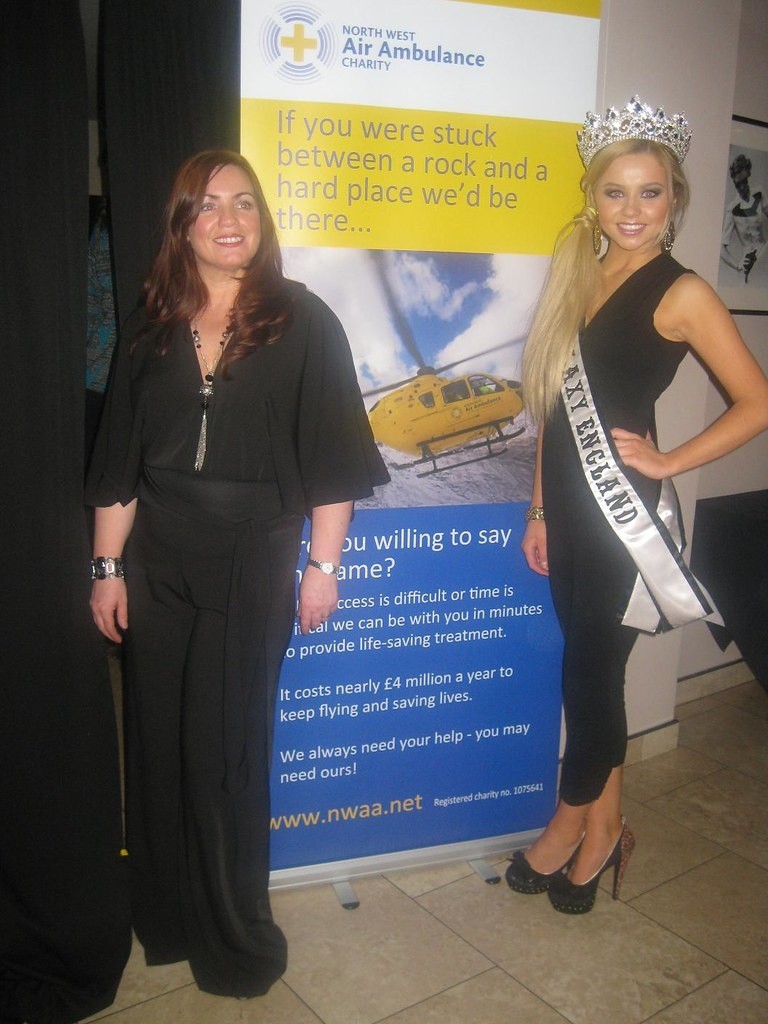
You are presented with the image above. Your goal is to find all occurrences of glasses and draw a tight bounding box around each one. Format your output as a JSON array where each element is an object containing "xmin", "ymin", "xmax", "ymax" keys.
[{"xmin": 734, "ymin": 175, "xmax": 748, "ymax": 188}]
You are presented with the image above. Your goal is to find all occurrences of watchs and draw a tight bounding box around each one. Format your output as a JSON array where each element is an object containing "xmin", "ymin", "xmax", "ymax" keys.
[{"xmin": 307, "ymin": 557, "xmax": 340, "ymax": 574}]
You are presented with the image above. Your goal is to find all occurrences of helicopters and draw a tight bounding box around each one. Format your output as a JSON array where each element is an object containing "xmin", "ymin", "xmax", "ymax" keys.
[{"xmin": 360, "ymin": 314, "xmax": 529, "ymax": 479}]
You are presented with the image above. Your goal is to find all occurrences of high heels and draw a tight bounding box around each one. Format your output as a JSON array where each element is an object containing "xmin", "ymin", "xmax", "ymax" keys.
[{"xmin": 505, "ymin": 815, "xmax": 636, "ymax": 915}]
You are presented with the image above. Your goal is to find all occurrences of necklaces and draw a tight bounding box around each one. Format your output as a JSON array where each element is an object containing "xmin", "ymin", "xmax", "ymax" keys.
[{"xmin": 190, "ymin": 317, "xmax": 231, "ymax": 473}]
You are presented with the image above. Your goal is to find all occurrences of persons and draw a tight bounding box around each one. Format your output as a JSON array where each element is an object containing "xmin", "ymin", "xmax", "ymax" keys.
[
  {"xmin": 83, "ymin": 147, "xmax": 390, "ymax": 999},
  {"xmin": 721, "ymin": 153, "xmax": 768, "ymax": 282},
  {"xmin": 506, "ymin": 138, "xmax": 768, "ymax": 915}
]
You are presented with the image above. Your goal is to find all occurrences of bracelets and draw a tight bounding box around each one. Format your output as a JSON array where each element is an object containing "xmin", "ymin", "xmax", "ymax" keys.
[
  {"xmin": 524, "ymin": 505, "xmax": 545, "ymax": 521},
  {"xmin": 88, "ymin": 556, "xmax": 126, "ymax": 580}
]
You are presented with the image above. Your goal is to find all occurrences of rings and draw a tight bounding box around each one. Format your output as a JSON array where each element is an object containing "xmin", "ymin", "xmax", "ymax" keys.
[{"xmin": 321, "ymin": 615, "xmax": 330, "ymax": 619}]
